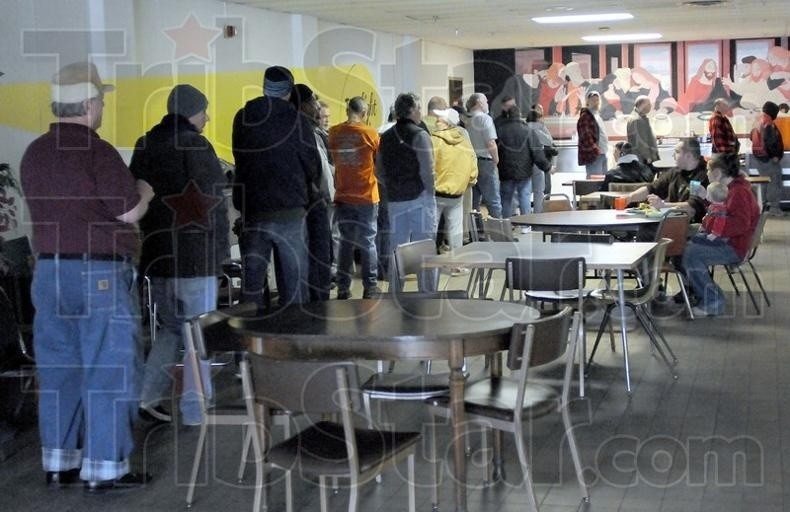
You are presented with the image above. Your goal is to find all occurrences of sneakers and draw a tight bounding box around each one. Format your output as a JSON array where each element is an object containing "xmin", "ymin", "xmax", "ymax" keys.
[
  {"xmin": 137, "ymin": 402, "xmax": 173, "ymax": 422},
  {"xmin": 338, "ymin": 292, "xmax": 352, "ymax": 299},
  {"xmin": 522, "ymin": 226, "xmax": 532, "ymax": 234},
  {"xmin": 632, "ymin": 284, "xmax": 665, "ymax": 297},
  {"xmin": 769, "ymin": 208, "xmax": 785, "ymax": 217},
  {"xmin": 674, "ymin": 285, "xmax": 696, "ymax": 302},
  {"xmin": 688, "ymin": 307, "xmax": 710, "ymax": 318},
  {"xmin": 364, "ymin": 287, "xmax": 382, "ymax": 298}
]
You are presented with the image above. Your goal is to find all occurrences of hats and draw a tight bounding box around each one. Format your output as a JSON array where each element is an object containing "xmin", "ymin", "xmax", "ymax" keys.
[
  {"xmin": 432, "ymin": 108, "xmax": 461, "ymax": 125},
  {"xmin": 168, "ymin": 85, "xmax": 209, "ymax": 119},
  {"xmin": 51, "ymin": 61, "xmax": 114, "ymax": 104},
  {"xmin": 263, "ymin": 66, "xmax": 294, "ymax": 97}
]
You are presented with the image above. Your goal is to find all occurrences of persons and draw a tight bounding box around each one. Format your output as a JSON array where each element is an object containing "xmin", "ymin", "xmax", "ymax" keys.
[{"xmin": 20, "ymin": 60, "xmax": 786, "ymax": 499}]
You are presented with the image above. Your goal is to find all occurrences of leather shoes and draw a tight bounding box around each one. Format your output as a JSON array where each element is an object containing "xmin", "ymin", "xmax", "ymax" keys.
[
  {"xmin": 84, "ymin": 471, "xmax": 155, "ymax": 497},
  {"xmin": 47, "ymin": 468, "xmax": 87, "ymax": 488}
]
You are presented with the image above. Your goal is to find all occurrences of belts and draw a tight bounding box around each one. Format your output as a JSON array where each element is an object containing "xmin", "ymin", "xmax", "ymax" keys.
[{"xmin": 38, "ymin": 252, "xmax": 123, "ymax": 261}]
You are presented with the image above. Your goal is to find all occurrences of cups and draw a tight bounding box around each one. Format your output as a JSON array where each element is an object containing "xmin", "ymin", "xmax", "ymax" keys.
[
  {"xmin": 690, "ymin": 181, "xmax": 701, "ymax": 196},
  {"xmin": 615, "ymin": 197, "xmax": 625, "ymax": 210}
]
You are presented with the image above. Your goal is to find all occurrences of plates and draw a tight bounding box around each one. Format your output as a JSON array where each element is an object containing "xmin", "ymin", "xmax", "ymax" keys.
[{"xmin": 627, "ymin": 208, "xmax": 656, "ymax": 212}]
